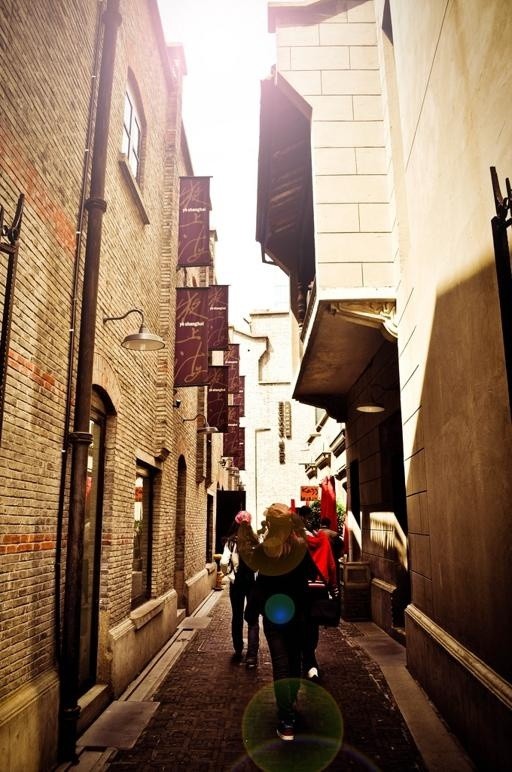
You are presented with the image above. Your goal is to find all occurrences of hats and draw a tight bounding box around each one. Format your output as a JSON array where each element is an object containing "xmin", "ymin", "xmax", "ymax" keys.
[{"xmin": 233, "ymin": 510, "xmax": 254, "ymax": 528}]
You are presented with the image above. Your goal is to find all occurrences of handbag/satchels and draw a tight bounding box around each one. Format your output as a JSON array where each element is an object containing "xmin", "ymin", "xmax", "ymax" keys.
[{"xmin": 304, "ymin": 579, "xmax": 330, "ymax": 624}]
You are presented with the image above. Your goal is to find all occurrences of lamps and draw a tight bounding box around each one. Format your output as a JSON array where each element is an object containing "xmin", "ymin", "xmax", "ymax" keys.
[
  {"xmin": 103, "ymin": 309, "xmax": 166, "ymax": 352},
  {"xmin": 183, "ymin": 413, "xmax": 219, "ymax": 434},
  {"xmin": 221, "ymin": 458, "xmax": 240, "ymax": 480},
  {"xmin": 174, "ymin": 399, "xmax": 182, "ymax": 408}
]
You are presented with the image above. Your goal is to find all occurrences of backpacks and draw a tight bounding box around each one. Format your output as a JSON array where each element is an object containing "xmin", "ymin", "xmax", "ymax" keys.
[{"xmin": 232, "ymin": 535, "xmax": 256, "ymax": 596}]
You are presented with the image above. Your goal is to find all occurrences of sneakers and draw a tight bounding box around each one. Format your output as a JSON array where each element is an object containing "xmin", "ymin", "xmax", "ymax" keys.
[
  {"xmin": 274, "ymin": 720, "xmax": 299, "ymax": 743},
  {"xmin": 305, "ymin": 665, "xmax": 320, "ymax": 681},
  {"xmin": 245, "ymin": 655, "xmax": 259, "ymax": 671}
]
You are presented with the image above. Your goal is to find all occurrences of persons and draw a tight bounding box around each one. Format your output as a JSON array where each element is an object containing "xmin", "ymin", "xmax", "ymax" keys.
[{"xmin": 218, "ymin": 500, "xmax": 346, "ymax": 742}]
